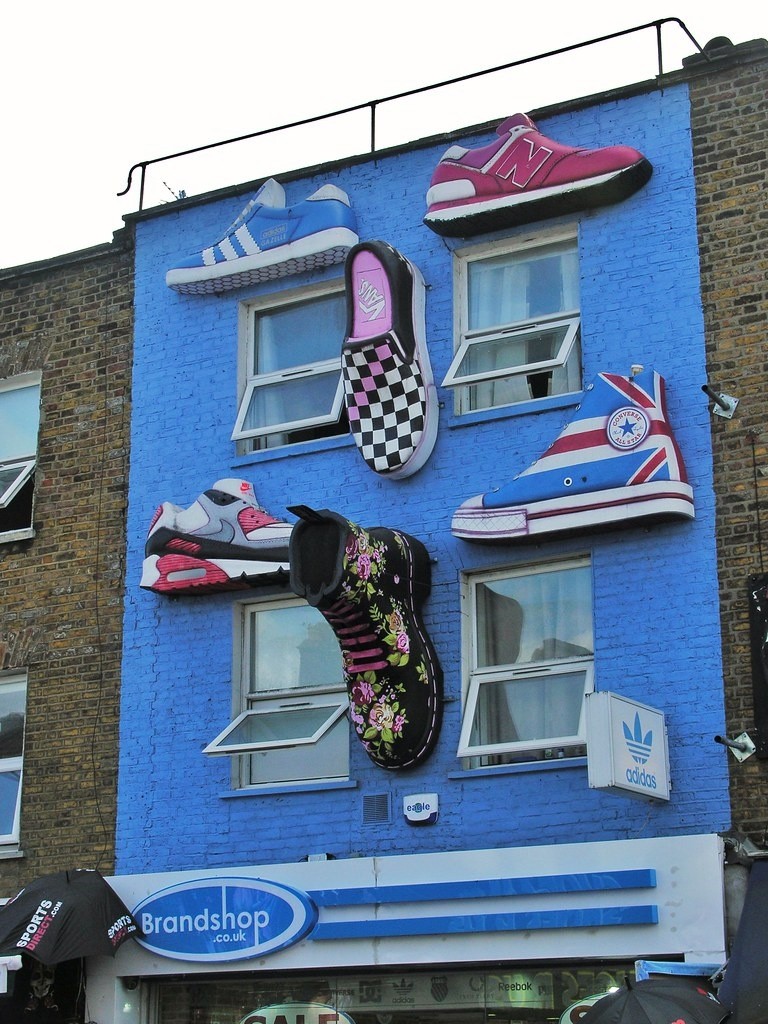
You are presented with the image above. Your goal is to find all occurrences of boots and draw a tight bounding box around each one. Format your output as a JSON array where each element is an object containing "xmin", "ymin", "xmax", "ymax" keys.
[{"xmin": 285, "ymin": 505, "xmax": 444, "ymax": 772}]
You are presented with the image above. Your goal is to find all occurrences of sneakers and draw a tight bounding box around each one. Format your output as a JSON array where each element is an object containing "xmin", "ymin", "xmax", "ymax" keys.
[
  {"xmin": 423, "ymin": 112, "xmax": 654, "ymax": 238},
  {"xmin": 450, "ymin": 373, "xmax": 696, "ymax": 540},
  {"xmin": 341, "ymin": 241, "xmax": 440, "ymax": 479},
  {"xmin": 166, "ymin": 177, "xmax": 358, "ymax": 293},
  {"xmin": 139, "ymin": 478, "xmax": 296, "ymax": 593}
]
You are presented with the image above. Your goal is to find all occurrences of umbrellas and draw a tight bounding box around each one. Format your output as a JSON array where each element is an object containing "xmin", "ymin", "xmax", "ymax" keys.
[
  {"xmin": 577, "ymin": 976, "xmax": 733, "ymax": 1024},
  {"xmin": 0, "ymin": 870, "xmax": 147, "ymax": 968}
]
